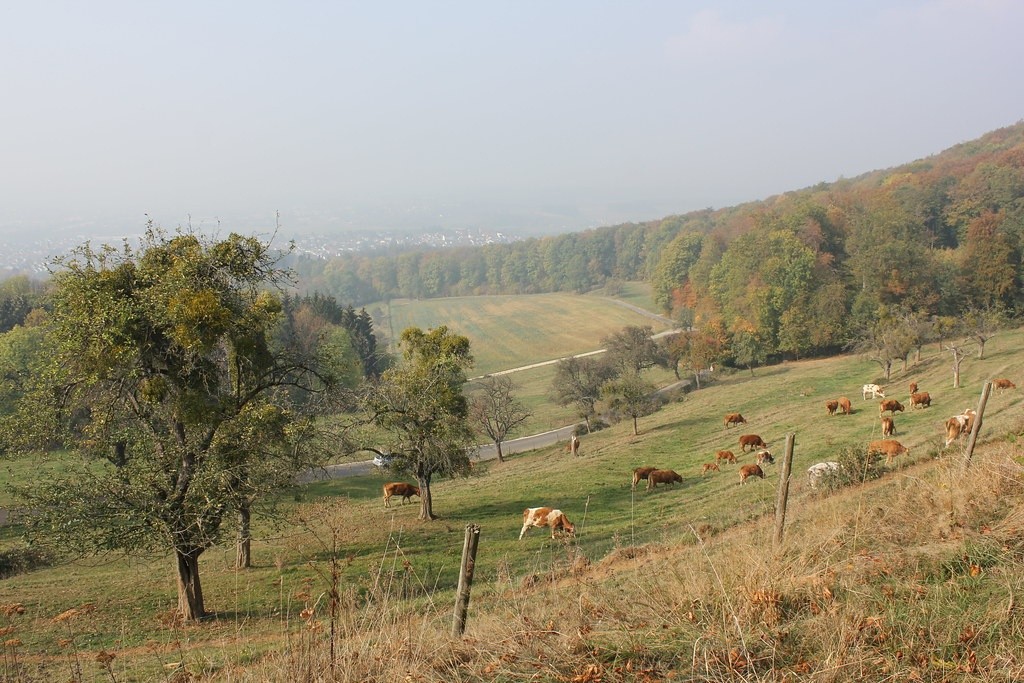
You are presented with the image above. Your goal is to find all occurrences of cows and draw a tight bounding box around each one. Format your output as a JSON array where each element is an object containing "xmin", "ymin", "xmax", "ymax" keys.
[
  {"xmin": 648, "ymin": 470, "xmax": 682, "ymax": 491},
  {"xmin": 632, "ymin": 467, "xmax": 659, "ymax": 490},
  {"xmin": 564, "ymin": 439, "xmax": 580, "ymax": 452},
  {"xmin": 826, "ymin": 379, "xmax": 1016, "ymax": 464},
  {"xmin": 519, "ymin": 507, "xmax": 576, "ymax": 540},
  {"xmin": 702, "ymin": 413, "xmax": 774, "ymax": 486},
  {"xmin": 383, "ymin": 481, "xmax": 420, "ymax": 508}
]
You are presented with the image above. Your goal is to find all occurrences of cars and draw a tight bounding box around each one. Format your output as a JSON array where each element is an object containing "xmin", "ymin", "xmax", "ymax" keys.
[{"xmin": 373, "ymin": 449, "xmax": 404, "ymax": 470}]
